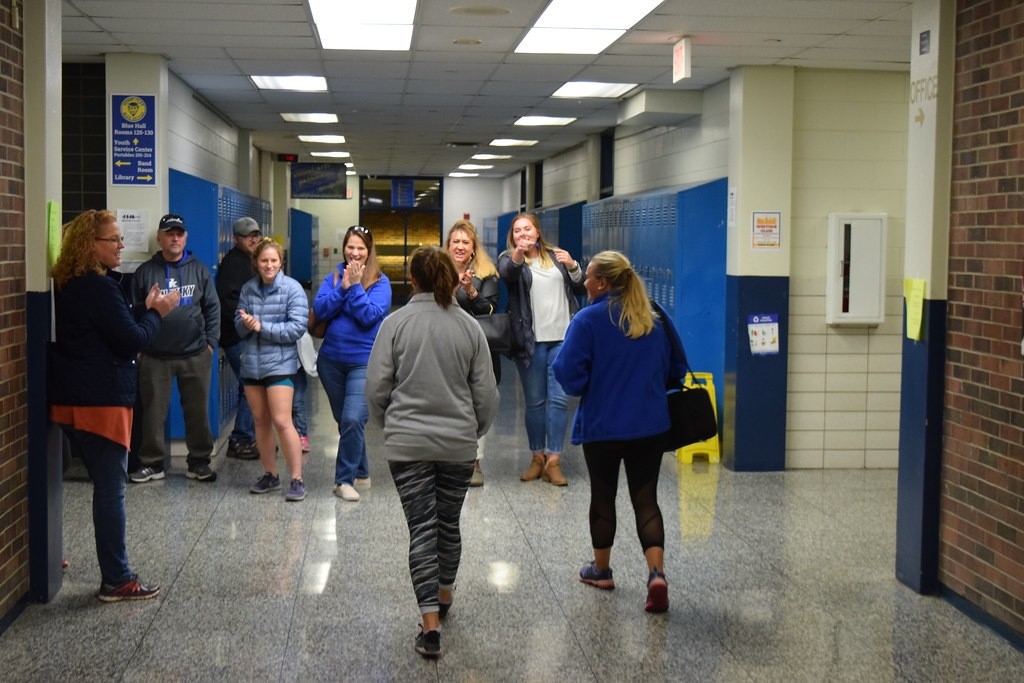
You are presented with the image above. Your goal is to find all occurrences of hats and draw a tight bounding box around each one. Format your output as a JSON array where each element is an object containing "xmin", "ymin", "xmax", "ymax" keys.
[
  {"xmin": 159, "ymin": 214, "xmax": 186, "ymax": 232},
  {"xmin": 233, "ymin": 217, "xmax": 261, "ymax": 236}
]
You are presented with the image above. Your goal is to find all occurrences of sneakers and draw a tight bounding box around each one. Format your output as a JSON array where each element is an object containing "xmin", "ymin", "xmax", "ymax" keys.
[
  {"xmin": 226, "ymin": 440, "xmax": 258, "ymax": 458},
  {"xmin": 185, "ymin": 461, "xmax": 216, "ymax": 481},
  {"xmin": 130, "ymin": 465, "xmax": 165, "ymax": 482},
  {"xmin": 98, "ymin": 574, "xmax": 159, "ymax": 601},
  {"xmin": 249, "ymin": 472, "xmax": 281, "ymax": 493},
  {"xmin": 579, "ymin": 561, "xmax": 614, "ymax": 588},
  {"xmin": 438, "ymin": 600, "xmax": 452, "ymax": 618},
  {"xmin": 413, "ymin": 623, "xmax": 442, "ymax": 656},
  {"xmin": 644, "ymin": 566, "xmax": 669, "ymax": 613},
  {"xmin": 285, "ymin": 475, "xmax": 306, "ymax": 501}
]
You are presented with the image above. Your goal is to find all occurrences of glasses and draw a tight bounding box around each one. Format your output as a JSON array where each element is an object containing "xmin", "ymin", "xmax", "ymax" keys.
[
  {"xmin": 237, "ymin": 234, "xmax": 262, "ymax": 241},
  {"xmin": 349, "ymin": 226, "xmax": 369, "ymax": 235},
  {"xmin": 95, "ymin": 237, "xmax": 124, "ymax": 244}
]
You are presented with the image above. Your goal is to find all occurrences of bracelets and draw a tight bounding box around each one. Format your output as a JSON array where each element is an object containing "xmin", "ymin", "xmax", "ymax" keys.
[
  {"xmin": 568, "ymin": 260, "xmax": 578, "ymax": 272},
  {"xmin": 466, "ymin": 288, "xmax": 476, "ymax": 297}
]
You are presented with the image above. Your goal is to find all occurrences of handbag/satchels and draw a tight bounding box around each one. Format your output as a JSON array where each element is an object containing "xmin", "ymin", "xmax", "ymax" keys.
[
  {"xmin": 307, "ymin": 271, "xmax": 338, "ymax": 337},
  {"xmin": 661, "ymin": 385, "xmax": 718, "ymax": 453},
  {"xmin": 470, "ymin": 304, "xmax": 511, "ymax": 352}
]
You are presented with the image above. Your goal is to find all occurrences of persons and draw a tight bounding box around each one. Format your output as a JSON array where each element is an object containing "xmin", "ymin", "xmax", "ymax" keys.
[
  {"xmin": 235, "ymin": 236, "xmax": 309, "ymax": 502},
  {"xmin": 48, "ymin": 209, "xmax": 180, "ymax": 603},
  {"xmin": 553, "ymin": 250, "xmax": 688, "ymax": 613},
  {"xmin": 313, "ymin": 225, "xmax": 392, "ymax": 502},
  {"xmin": 445, "ymin": 222, "xmax": 501, "ymax": 488},
  {"xmin": 128, "ymin": 214, "xmax": 222, "ymax": 483},
  {"xmin": 215, "ymin": 217, "xmax": 279, "ymax": 461},
  {"xmin": 366, "ymin": 244, "xmax": 502, "ymax": 657},
  {"xmin": 291, "ymin": 329, "xmax": 318, "ymax": 452},
  {"xmin": 497, "ymin": 211, "xmax": 588, "ymax": 486}
]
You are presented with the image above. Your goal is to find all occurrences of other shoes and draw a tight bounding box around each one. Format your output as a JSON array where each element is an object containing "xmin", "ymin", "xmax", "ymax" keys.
[
  {"xmin": 541, "ymin": 460, "xmax": 568, "ymax": 485},
  {"xmin": 300, "ymin": 436, "xmax": 310, "ymax": 451},
  {"xmin": 333, "ymin": 477, "xmax": 371, "ymax": 492},
  {"xmin": 520, "ymin": 455, "xmax": 547, "ymax": 481},
  {"xmin": 469, "ymin": 470, "xmax": 483, "ymax": 486},
  {"xmin": 335, "ymin": 483, "xmax": 360, "ymax": 500}
]
